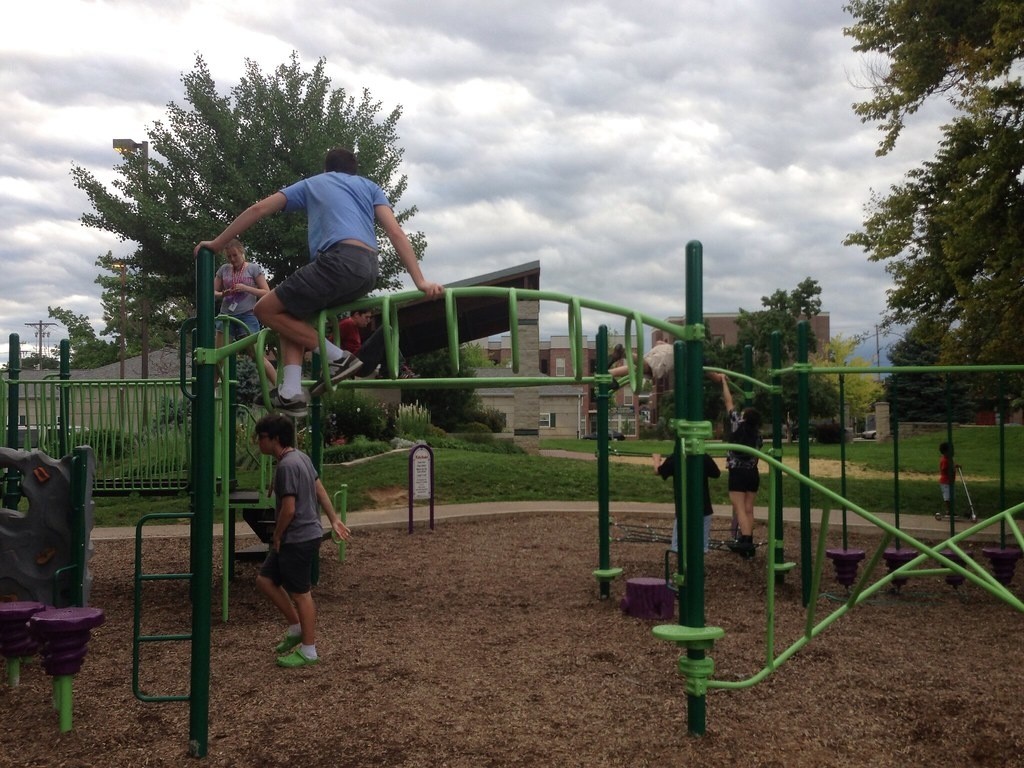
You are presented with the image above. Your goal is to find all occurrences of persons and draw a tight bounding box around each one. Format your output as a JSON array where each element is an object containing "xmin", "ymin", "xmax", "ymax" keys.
[
  {"xmin": 722, "ymin": 374, "xmax": 762, "ymax": 555},
  {"xmin": 255, "ymin": 414, "xmax": 352, "ymax": 667},
  {"xmin": 328, "ymin": 310, "xmax": 421, "ymax": 379},
  {"xmin": 215, "ymin": 240, "xmax": 276, "ymax": 387},
  {"xmin": 652, "ymin": 438, "xmax": 720, "ymax": 553},
  {"xmin": 194, "ymin": 148, "xmax": 443, "ymax": 417},
  {"xmin": 939, "ymin": 443, "xmax": 962, "ymax": 517},
  {"xmin": 609, "ymin": 325, "xmax": 724, "ymax": 391}
]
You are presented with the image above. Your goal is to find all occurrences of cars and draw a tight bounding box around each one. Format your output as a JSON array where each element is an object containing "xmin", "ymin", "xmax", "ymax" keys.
[
  {"xmin": 861, "ymin": 429, "xmax": 877, "ymax": 440},
  {"xmin": 582, "ymin": 428, "xmax": 626, "ymax": 442}
]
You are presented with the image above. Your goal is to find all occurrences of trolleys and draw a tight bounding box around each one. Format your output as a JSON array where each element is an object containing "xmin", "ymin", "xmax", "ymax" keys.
[{"xmin": 934, "ymin": 466, "xmax": 978, "ymax": 525}]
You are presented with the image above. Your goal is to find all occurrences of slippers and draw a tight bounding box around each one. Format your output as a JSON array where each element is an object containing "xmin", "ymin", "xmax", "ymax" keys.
[
  {"xmin": 275, "ymin": 648, "xmax": 318, "ymax": 667},
  {"xmin": 276, "ymin": 633, "xmax": 301, "ymax": 653}
]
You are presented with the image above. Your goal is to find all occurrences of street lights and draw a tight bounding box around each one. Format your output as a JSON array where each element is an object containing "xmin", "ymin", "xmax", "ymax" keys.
[
  {"xmin": 113, "ymin": 137, "xmax": 150, "ymax": 434},
  {"xmin": 110, "ymin": 262, "xmax": 127, "ymax": 428}
]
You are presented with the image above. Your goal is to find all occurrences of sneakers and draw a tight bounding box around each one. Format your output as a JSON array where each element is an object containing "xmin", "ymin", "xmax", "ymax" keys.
[
  {"xmin": 945, "ymin": 511, "xmax": 960, "ymax": 517},
  {"xmin": 253, "ymin": 388, "xmax": 307, "ymax": 417},
  {"xmin": 727, "ymin": 534, "xmax": 754, "ymax": 551},
  {"xmin": 309, "ymin": 350, "xmax": 363, "ymax": 395},
  {"xmin": 376, "ymin": 372, "xmax": 387, "ymax": 379},
  {"xmin": 399, "ymin": 369, "xmax": 420, "ymax": 378}
]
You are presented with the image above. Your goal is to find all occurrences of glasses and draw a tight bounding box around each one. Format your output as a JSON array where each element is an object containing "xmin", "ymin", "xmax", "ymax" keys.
[{"xmin": 258, "ymin": 434, "xmax": 268, "ymax": 440}]
